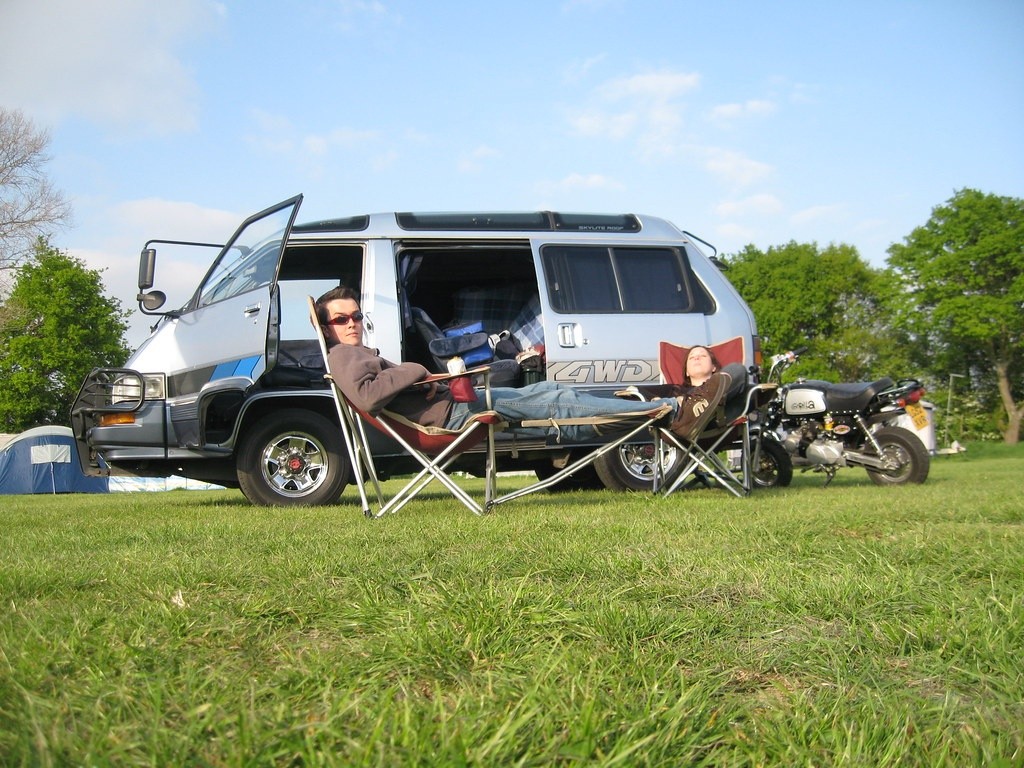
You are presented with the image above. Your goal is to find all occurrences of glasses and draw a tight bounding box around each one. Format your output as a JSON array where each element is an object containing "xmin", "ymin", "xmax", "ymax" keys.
[{"xmin": 323, "ymin": 313, "xmax": 363, "ymax": 325}]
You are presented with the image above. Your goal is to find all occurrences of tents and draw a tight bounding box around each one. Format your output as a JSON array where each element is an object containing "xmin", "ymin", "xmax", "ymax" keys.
[{"xmin": 0, "ymin": 424, "xmax": 111, "ymax": 495}]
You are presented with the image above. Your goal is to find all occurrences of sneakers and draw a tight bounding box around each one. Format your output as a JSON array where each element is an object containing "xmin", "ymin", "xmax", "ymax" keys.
[{"xmin": 670, "ymin": 372, "xmax": 726, "ymax": 441}]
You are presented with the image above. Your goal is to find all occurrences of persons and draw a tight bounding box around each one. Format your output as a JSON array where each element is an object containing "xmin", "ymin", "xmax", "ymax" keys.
[
  {"xmin": 614, "ymin": 344, "xmax": 748, "ymax": 428},
  {"xmin": 315, "ymin": 287, "xmax": 732, "ymax": 440}
]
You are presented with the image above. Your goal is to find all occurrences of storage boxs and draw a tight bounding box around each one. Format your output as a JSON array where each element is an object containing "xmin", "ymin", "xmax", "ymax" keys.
[{"xmin": 444, "ymin": 318, "xmax": 494, "ymax": 370}]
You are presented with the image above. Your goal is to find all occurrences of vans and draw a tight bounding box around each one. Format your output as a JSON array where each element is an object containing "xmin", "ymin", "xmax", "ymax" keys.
[{"xmin": 70, "ymin": 192, "xmax": 765, "ymax": 509}]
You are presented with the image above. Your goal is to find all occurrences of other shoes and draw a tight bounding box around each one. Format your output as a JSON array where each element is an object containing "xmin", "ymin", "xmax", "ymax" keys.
[{"xmin": 713, "ymin": 371, "xmax": 732, "ymax": 395}]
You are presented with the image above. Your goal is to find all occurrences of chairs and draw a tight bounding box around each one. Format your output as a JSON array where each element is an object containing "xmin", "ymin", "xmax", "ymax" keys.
[
  {"xmin": 614, "ymin": 335, "xmax": 778, "ymax": 495},
  {"xmin": 411, "ymin": 306, "xmax": 522, "ymax": 389},
  {"xmin": 307, "ymin": 295, "xmax": 672, "ymax": 520}
]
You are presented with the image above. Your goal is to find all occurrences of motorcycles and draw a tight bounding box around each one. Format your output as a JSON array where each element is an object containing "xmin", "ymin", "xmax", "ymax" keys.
[{"xmin": 731, "ymin": 345, "xmax": 931, "ymax": 487}]
